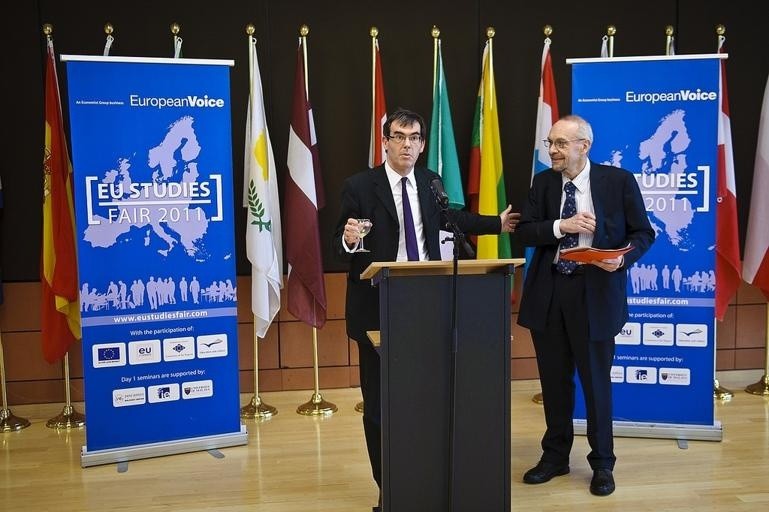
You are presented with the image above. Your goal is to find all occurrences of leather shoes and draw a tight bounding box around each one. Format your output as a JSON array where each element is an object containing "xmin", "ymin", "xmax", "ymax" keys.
[
  {"xmin": 589, "ymin": 467, "xmax": 614, "ymax": 496},
  {"xmin": 523, "ymin": 460, "xmax": 570, "ymax": 484}
]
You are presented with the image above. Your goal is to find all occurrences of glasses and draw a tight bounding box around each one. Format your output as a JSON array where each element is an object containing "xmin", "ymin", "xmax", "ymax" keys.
[
  {"xmin": 542, "ymin": 138, "xmax": 584, "ymax": 148},
  {"xmin": 386, "ymin": 133, "xmax": 422, "ymax": 143}
]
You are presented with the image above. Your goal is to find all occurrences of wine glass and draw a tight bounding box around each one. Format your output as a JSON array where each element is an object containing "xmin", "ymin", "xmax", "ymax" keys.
[{"xmin": 354, "ymin": 218, "xmax": 371, "ymax": 252}]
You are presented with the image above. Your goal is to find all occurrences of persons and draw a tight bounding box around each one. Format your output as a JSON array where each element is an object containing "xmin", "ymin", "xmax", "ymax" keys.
[
  {"xmin": 514, "ymin": 115, "xmax": 655, "ymax": 495},
  {"xmin": 329, "ymin": 110, "xmax": 521, "ymax": 512}
]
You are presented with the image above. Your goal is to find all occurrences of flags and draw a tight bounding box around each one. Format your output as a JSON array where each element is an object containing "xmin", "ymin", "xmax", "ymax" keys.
[
  {"xmin": 466, "ymin": 40, "xmax": 512, "ymax": 260},
  {"xmin": 366, "ymin": 38, "xmax": 388, "ymax": 169},
  {"xmin": 39, "ymin": 43, "xmax": 84, "ymax": 366},
  {"xmin": 601, "ymin": 39, "xmax": 610, "ymax": 58},
  {"xmin": 741, "ymin": 76, "xmax": 769, "ymax": 302},
  {"xmin": 242, "ymin": 39, "xmax": 285, "ymax": 339},
  {"xmin": 714, "ymin": 36, "xmax": 743, "ymax": 323},
  {"xmin": 427, "ymin": 40, "xmax": 466, "ymax": 211},
  {"xmin": 523, "ymin": 38, "xmax": 559, "ymax": 282},
  {"xmin": 666, "ymin": 37, "xmax": 674, "ymax": 56},
  {"xmin": 281, "ymin": 36, "xmax": 328, "ymax": 328}
]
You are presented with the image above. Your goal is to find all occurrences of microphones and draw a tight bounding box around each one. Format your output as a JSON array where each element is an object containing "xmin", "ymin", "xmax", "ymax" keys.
[{"xmin": 425, "ymin": 172, "xmax": 449, "ymax": 209}]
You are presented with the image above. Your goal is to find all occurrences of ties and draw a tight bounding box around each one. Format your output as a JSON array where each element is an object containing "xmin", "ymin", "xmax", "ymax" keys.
[
  {"xmin": 556, "ymin": 181, "xmax": 578, "ymax": 275},
  {"xmin": 400, "ymin": 177, "xmax": 420, "ymax": 262}
]
